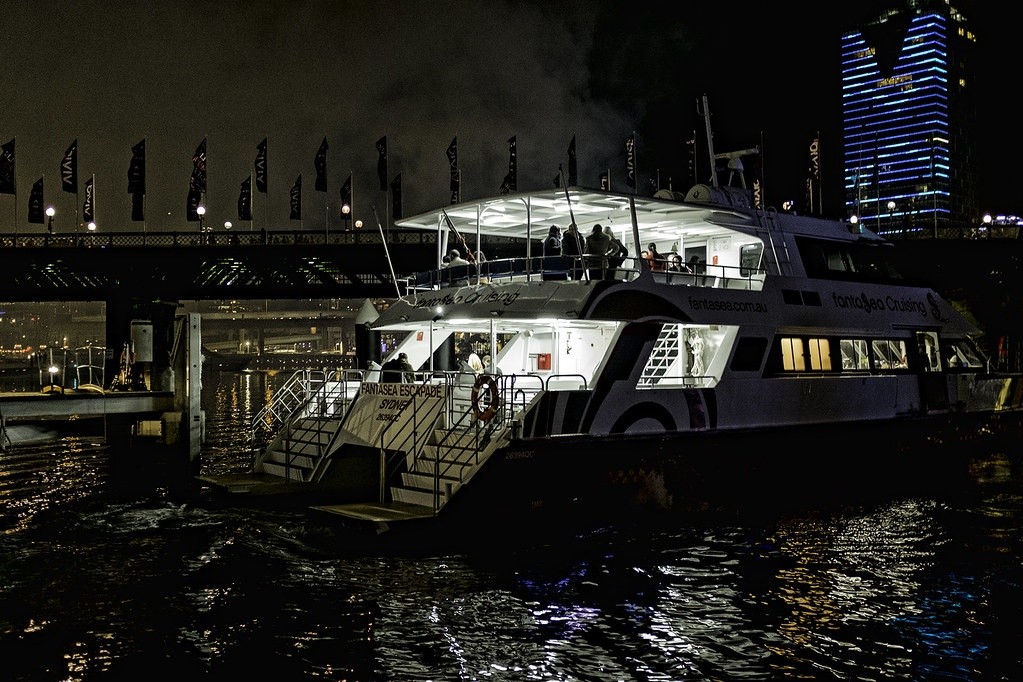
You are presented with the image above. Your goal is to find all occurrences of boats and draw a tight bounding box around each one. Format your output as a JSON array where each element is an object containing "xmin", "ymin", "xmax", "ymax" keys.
[{"xmin": 295, "ymin": 164, "xmax": 1023, "ymax": 538}]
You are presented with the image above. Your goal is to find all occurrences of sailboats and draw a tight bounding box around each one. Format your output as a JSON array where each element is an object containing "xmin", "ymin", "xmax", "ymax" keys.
[{"xmin": 36, "ymin": 340, "xmax": 107, "ymax": 395}]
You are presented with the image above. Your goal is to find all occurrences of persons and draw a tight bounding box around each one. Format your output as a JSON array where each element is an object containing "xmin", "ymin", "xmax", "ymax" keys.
[
  {"xmin": 642, "ymin": 242, "xmax": 699, "ymax": 272},
  {"xmin": 458, "ymin": 342, "xmax": 483, "ymax": 371},
  {"xmin": 396, "ymin": 353, "xmax": 417, "ymax": 383},
  {"xmin": 442, "ymin": 250, "xmax": 486, "ymax": 269},
  {"xmin": 543, "ymin": 224, "xmax": 628, "ymax": 281}
]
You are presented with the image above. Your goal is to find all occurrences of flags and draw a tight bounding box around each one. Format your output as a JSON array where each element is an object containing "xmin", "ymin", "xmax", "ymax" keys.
[
  {"xmin": 624, "ymin": 133, "xmax": 635, "ymax": 189},
  {"xmin": 290, "ymin": 176, "xmax": 300, "ymax": 220},
  {"xmin": 314, "ymin": 137, "xmax": 329, "ymax": 192},
  {"xmin": 598, "ymin": 172, "xmax": 608, "ymax": 191},
  {"xmin": 340, "ymin": 175, "xmax": 352, "ymax": 220},
  {"xmin": 238, "ymin": 176, "xmax": 252, "ymax": 221},
  {"xmin": 686, "ymin": 137, "xmax": 695, "ymax": 176},
  {"xmin": 567, "ymin": 135, "xmax": 577, "ymax": 186},
  {"xmin": 83, "ymin": 177, "xmax": 94, "ymax": 222},
  {"xmin": 390, "ymin": 174, "xmax": 402, "ymax": 220},
  {"xmin": 254, "ymin": 138, "xmax": 266, "ymax": 193},
  {"xmin": 753, "ymin": 178, "xmax": 761, "ymax": 208},
  {"xmin": 28, "ymin": 178, "xmax": 44, "ymax": 224},
  {"xmin": 446, "ymin": 137, "xmax": 459, "ymax": 204},
  {"xmin": 806, "ymin": 134, "xmax": 821, "ymax": 206},
  {"xmin": 0, "ymin": 139, "xmax": 16, "ymax": 195},
  {"xmin": 127, "ymin": 138, "xmax": 146, "ymax": 222},
  {"xmin": 187, "ymin": 138, "xmax": 207, "ymax": 222},
  {"xmin": 61, "ymin": 140, "xmax": 77, "ymax": 193},
  {"xmin": 501, "ymin": 135, "xmax": 517, "ymax": 195},
  {"xmin": 376, "ymin": 135, "xmax": 387, "ymax": 192}
]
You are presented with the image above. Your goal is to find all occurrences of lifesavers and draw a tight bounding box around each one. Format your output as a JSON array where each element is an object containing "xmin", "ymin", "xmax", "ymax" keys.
[{"xmin": 470, "ymin": 375, "xmax": 500, "ymax": 419}]
